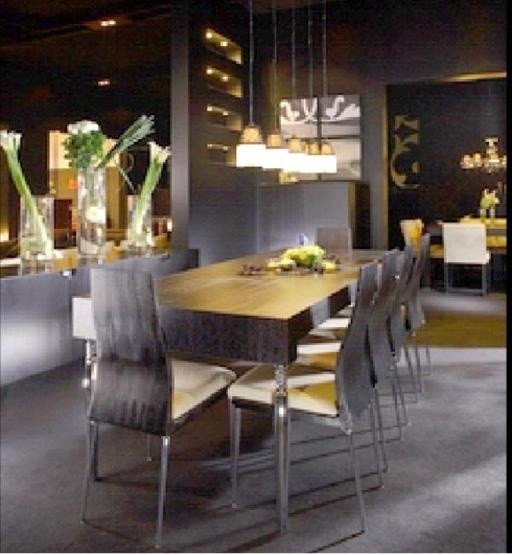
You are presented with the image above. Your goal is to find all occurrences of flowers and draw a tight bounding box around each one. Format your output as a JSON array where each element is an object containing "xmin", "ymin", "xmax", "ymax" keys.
[
  {"xmin": 0, "ymin": 130, "xmax": 41, "ymax": 220},
  {"xmin": 489, "ymin": 191, "xmax": 500, "ymax": 207},
  {"xmin": 59, "ymin": 113, "xmax": 156, "ymax": 207},
  {"xmin": 479, "ymin": 190, "xmax": 489, "ymax": 209},
  {"xmin": 131, "ymin": 141, "xmax": 172, "ymax": 234}
]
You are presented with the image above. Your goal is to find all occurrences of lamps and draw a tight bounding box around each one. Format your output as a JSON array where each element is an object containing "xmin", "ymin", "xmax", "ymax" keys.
[
  {"xmin": 235, "ymin": 0, "xmax": 337, "ymax": 174},
  {"xmin": 458, "ymin": 137, "xmax": 507, "ymax": 176}
]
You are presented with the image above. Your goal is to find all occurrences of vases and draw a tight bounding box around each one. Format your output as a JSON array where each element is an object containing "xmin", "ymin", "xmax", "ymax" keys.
[
  {"xmin": 489, "ymin": 208, "xmax": 496, "ymax": 223},
  {"xmin": 127, "ymin": 195, "xmax": 153, "ymax": 251},
  {"xmin": 479, "ymin": 209, "xmax": 486, "ymax": 221},
  {"xmin": 76, "ymin": 165, "xmax": 107, "ymax": 254},
  {"xmin": 18, "ymin": 193, "xmax": 54, "ymax": 262}
]
